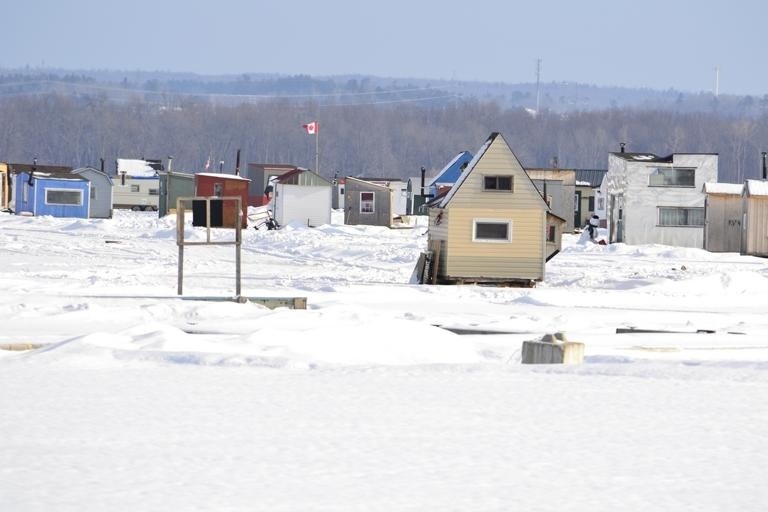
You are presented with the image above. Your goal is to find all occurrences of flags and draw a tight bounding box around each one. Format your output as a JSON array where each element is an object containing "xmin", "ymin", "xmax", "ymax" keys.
[{"xmin": 302, "ymin": 121, "xmax": 319, "ymax": 136}]
